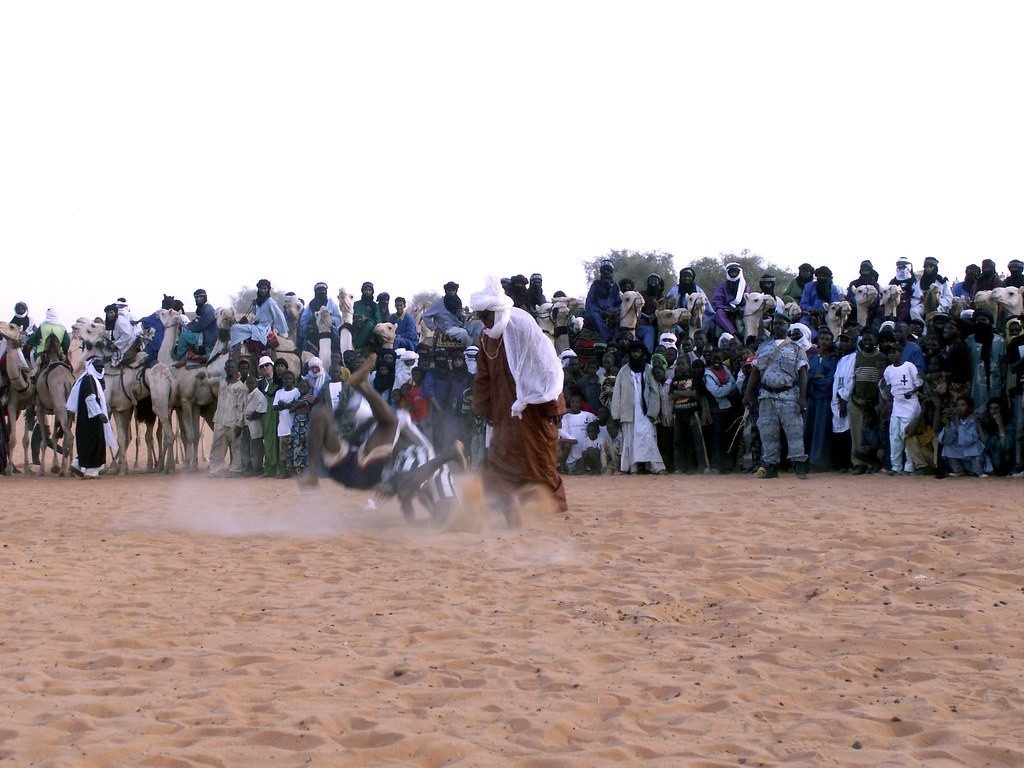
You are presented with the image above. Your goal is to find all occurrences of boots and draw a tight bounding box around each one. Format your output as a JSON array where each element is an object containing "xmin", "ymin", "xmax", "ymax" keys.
[
  {"xmin": 760, "ymin": 463, "xmax": 778, "ymax": 478},
  {"xmin": 792, "ymin": 460, "xmax": 807, "ymax": 479}
]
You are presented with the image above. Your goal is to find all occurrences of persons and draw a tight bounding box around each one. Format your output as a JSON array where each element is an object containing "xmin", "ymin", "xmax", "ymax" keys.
[{"xmin": 0, "ymin": 256, "xmax": 1024, "ymax": 526}]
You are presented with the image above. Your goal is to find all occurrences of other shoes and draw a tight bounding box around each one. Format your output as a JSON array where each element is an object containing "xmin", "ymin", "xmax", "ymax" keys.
[{"xmin": 630, "ymin": 457, "xmax": 1024, "ymax": 479}]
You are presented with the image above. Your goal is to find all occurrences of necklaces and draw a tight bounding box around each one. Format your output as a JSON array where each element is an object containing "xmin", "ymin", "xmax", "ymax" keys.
[{"xmin": 481, "ymin": 336, "xmax": 502, "ymax": 360}]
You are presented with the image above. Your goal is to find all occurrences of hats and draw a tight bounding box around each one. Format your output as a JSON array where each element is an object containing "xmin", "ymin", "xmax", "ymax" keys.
[
  {"xmin": 601, "ymin": 260, "xmax": 614, "ymax": 269},
  {"xmin": 774, "ymin": 312, "xmax": 793, "ymax": 324},
  {"xmin": 258, "ymin": 355, "xmax": 274, "ymax": 366},
  {"xmin": 530, "ymin": 273, "xmax": 542, "ymax": 282},
  {"xmin": 444, "ymin": 282, "xmax": 459, "ymax": 290},
  {"xmin": 884, "ymin": 344, "xmax": 902, "ymax": 352}
]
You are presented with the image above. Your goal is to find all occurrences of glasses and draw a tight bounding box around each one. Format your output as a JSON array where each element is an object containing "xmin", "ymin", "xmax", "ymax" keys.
[{"xmin": 260, "ymin": 362, "xmax": 271, "ymax": 368}]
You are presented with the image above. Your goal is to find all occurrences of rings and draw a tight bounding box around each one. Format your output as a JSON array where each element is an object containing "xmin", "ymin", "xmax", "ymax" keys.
[{"xmin": 804, "ymin": 408, "xmax": 807, "ymax": 410}]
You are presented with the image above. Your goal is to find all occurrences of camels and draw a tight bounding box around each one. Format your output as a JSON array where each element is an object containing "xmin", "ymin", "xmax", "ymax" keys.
[
  {"xmin": 406, "ymin": 290, "xmax": 710, "ymax": 370},
  {"xmin": 743, "ymin": 281, "xmax": 1024, "ymax": 349},
  {"xmin": 0, "ymin": 286, "xmax": 419, "ymax": 480}
]
